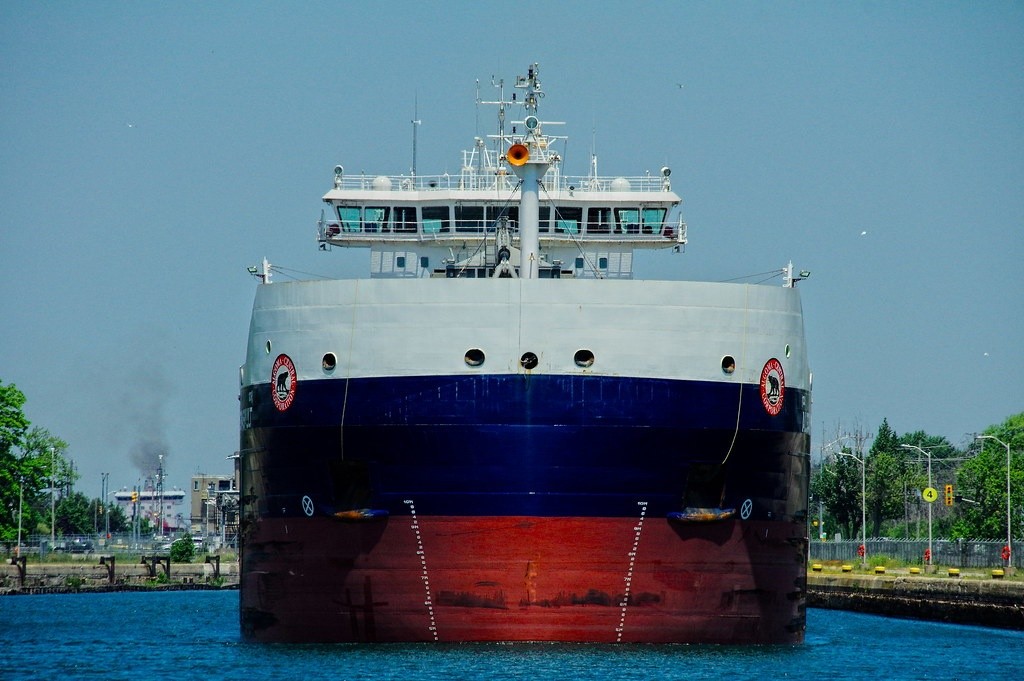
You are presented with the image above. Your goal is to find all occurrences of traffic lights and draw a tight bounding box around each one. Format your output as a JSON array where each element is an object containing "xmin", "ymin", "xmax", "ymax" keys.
[
  {"xmin": 839, "ymin": 452, "xmax": 867, "ymax": 564},
  {"xmin": 99, "ymin": 505, "xmax": 102, "ymax": 515},
  {"xmin": 944, "ymin": 484, "xmax": 954, "ymax": 507},
  {"xmin": 132, "ymin": 492, "xmax": 138, "ymax": 502},
  {"xmin": 899, "ymin": 443, "xmax": 934, "ymax": 566}
]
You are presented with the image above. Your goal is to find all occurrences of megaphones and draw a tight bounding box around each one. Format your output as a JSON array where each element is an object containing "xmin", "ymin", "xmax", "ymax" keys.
[{"xmin": 508, "ymin": 144, "xmax": 530, "ymax": 166}]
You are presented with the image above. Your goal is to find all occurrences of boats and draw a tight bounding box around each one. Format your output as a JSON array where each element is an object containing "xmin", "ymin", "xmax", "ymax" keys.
[{"xmin": 232, "ymin": 61, "xmax": 815, "ymax": 649}]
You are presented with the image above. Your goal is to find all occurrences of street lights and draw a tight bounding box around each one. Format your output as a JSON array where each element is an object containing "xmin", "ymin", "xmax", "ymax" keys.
[
  {"xmin": 819, "ymin": 435, "xmax": 849, "ymax": 540},
  {"xmin": 205, "ymin": 489, "xmax": 209, "ymax": 538},
  {"xmin": 17, "ymin": 475, "xmax": 24, "ymax": 565},
  {"xmin": 977, "ymin": 436, "xmax": 1013, "ymax": 568},
  {"xmin": 49, "ymin": 447, "xmax": 55, "ymax": 554}
]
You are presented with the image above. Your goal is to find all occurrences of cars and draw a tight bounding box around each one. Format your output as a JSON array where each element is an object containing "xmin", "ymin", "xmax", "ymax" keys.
[
  {"xmin": 151, "ymin": 537, "xmax": 175, "ymax": 549},
  {"xmin": 161, "ymin": 537, "xmax": 204, "ymax": 552},
  {"xmin": 52, "ymin": 538, "xmax": 95, "ymax": 555}
]
